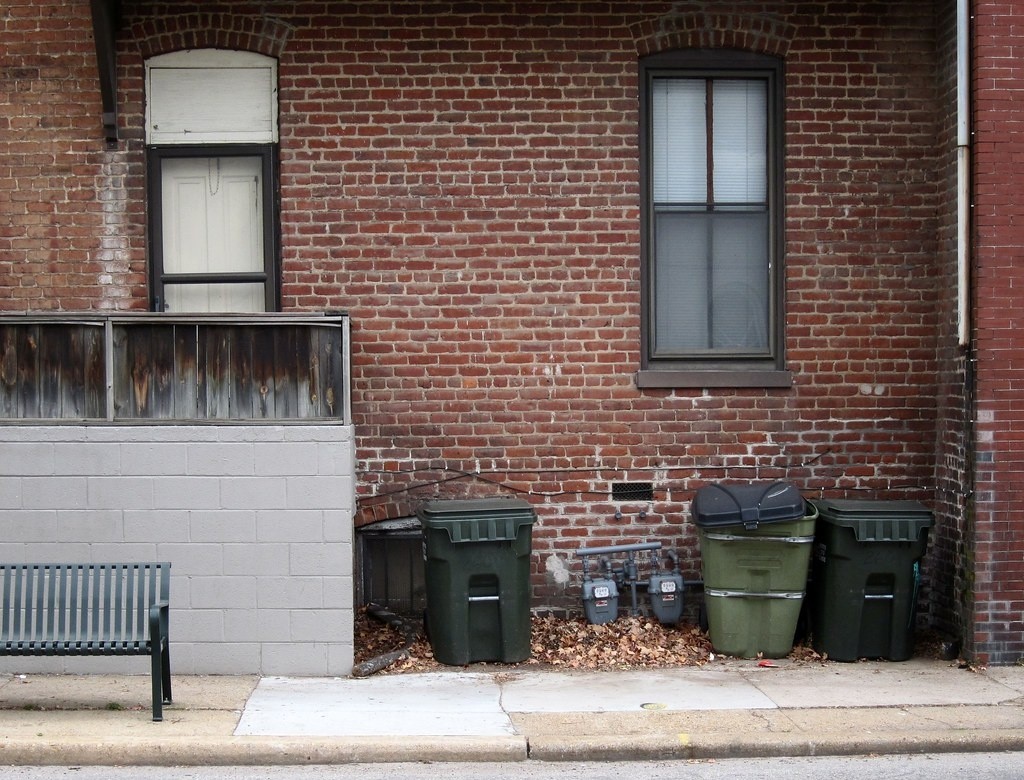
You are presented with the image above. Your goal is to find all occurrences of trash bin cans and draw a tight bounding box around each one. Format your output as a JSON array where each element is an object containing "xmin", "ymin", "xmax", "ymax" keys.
[
  {"xmin": 691, "ymin": 479, "xmax": 819, "ymax": 659},
  {"xmin": 812, "ymin": 496, "xmax": 936, "ymax": 663},
  {"xmin": 413, "ymin": 493, "xmax": 537, "ymax": 666}
]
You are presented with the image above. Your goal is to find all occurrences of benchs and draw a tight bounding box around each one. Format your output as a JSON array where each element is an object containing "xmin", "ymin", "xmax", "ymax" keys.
[{"xmin": 0, "ymin": 561, "xmax": 175, "ymax": 722}]
